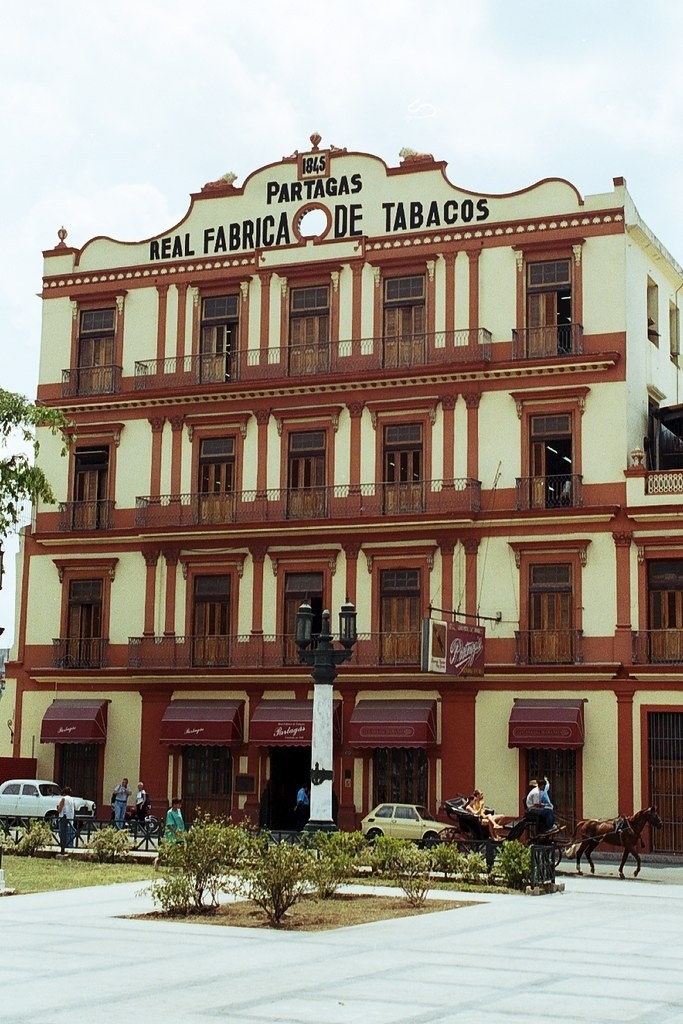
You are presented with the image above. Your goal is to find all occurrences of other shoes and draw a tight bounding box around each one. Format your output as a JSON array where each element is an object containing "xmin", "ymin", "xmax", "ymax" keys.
[
  {"xmin": 493, "ymin": 825, "xmax": 504, "ymax": 829},
  {"xmin": 546, "ymin": 825, "xmax": 558, "ymax": 833},
  {"xmin": 493, "ymin": 838, "xmax": 501, "ymax": 841}
]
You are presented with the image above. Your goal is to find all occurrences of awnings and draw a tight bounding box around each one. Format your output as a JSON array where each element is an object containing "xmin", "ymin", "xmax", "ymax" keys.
[
  {"xmin": 159, "ymin": 699, "xmax": 245, "ymax": 746},
  {"xmin": 349, "ymin": 699, "xmax": 438, "ymax": 749},
  {"xmin": 508, "ymin": 698, "xmax": 585, "ymax": 750},
  {"xmin": 249, "ymin": 699, "xmax": 342, "ymax": 748},
  {"xmin": 39, "ymin": 700, "xmax": 107, "ymax": 744}
]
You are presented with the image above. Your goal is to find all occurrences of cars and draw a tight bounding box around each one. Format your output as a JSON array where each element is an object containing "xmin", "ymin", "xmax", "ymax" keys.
[
  {"xmin": 360, "ymin": 803, "xmax": 457, "ymax": 849},
  {"xmin": 0, "ymin": 778, "xmax": 96, "ymax": 830}
]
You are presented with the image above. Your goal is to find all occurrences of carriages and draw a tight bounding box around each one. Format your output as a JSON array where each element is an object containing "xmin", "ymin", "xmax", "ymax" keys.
[{"xmin": 436, "ymin": 793, "xmax": 662, "ymax": 880}]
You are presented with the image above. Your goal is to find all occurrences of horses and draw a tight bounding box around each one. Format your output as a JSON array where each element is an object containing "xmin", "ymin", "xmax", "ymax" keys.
[{"xmin": 570, "ymin": 806, "xmax": 663, "ymax": 880}]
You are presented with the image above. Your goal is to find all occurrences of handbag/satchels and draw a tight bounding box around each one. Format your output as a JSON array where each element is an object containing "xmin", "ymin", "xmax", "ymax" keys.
[
  {"xmin": 142, "ymin": 794, "xmax": 150, "ymax": 804},
  {"xmin": 111, "ymin": 785, "xmax": 121, "ymax": 803}
]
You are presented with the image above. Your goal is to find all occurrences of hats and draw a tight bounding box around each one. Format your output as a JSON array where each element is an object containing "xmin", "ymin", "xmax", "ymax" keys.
[
  {"xmin": 530, "ymin": 779, "xmax": 538, "ymax": 786},
  {"xmin": 172, "ymin": 797, "xmax": 182, "ymax": 803}
]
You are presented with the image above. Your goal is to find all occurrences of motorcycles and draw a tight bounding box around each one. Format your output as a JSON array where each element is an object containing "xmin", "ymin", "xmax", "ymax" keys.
[{"xmin": 110, "ymin": 801, "xmax": 159, "ymax": 833}]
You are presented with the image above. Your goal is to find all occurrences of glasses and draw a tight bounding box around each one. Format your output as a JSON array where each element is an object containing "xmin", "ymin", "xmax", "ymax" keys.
[
  {"xmin": 472, "ymin": 799, "xmax": 477, "ymax": 801},
  {"xmin": 478, "ymin": 793, "xmax": 483, "ymax": 795}
]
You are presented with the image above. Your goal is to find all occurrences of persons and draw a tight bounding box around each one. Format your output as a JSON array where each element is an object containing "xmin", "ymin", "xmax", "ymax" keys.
[
  {"xmin": 57, "ymin": 787, "xmax": 74, "ymax": 848},
  {"xmin": 112, "ymin": 778, "xmax": 132, "ymax": 829},
  {"xmin": 465, "ymin": 794, "xmax": 507, "ymax": 842},
  {"xmin": 294, "ymin": 783, "xmax": 310, "ymax": 831},
  {"xmin": 527, "ymin": 779, "xmax": 559, "ymax": 833},
  {"xmin": 472, "ymin": 790, "xmax": 504, "ymax": 824},
  {"xmin": 164, "ymin": 797, "xmax": 188, "ymax": 843},
  {"xmin": 134, "ymin": 782, "xmax": 147, "ymax": 831},
  {"xmin": 332, "ymin": 790, "xmax": 338, "ymax": 826},
  {"xmin": 522, "ymin": 775, "xmax": 554, "ymax": 810}
]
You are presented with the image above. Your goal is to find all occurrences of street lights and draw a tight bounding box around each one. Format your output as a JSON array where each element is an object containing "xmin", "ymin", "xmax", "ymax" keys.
[{"xmin": 294, "ymin": 590, "xmax": 357, "ymax": 847}]
